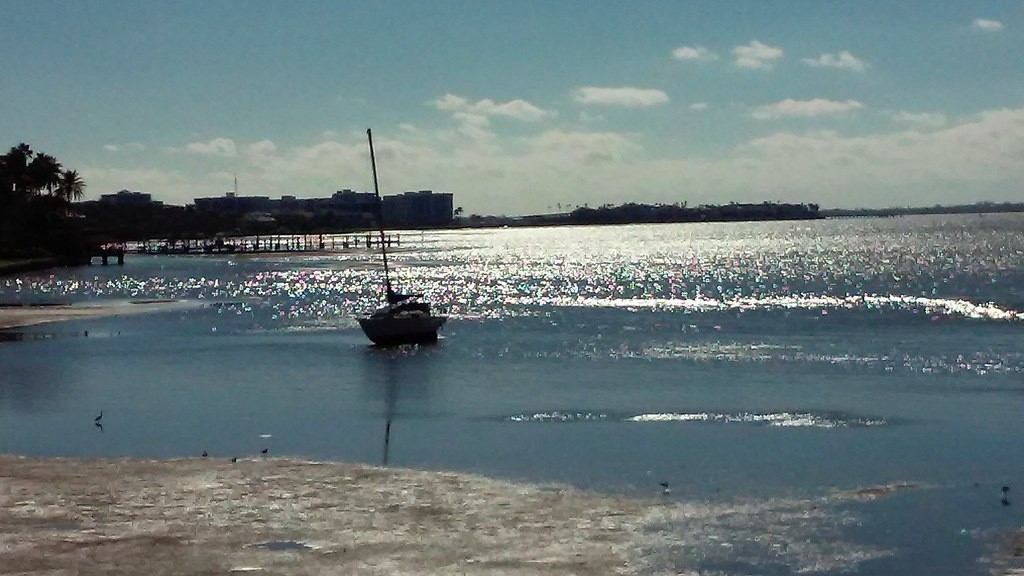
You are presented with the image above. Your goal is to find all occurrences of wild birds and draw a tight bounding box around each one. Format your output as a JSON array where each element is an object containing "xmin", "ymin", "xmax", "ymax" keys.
[
  {"xmin": 94, "ymin": 410, "xmax": 269, "ymax": 462},
  {"xmin": 1000, "ymin": 484, "xmax": 1012, "ymax": 498},
  {"xmin": 658, "ymin": 480, "xmax": 670, "ymax": 491}
]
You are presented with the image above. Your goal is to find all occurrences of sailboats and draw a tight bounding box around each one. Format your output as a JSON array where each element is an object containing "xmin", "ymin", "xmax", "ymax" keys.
[{"xmin": 353, "ymin": 128, "xmax": 450, "ymax": 346}]
